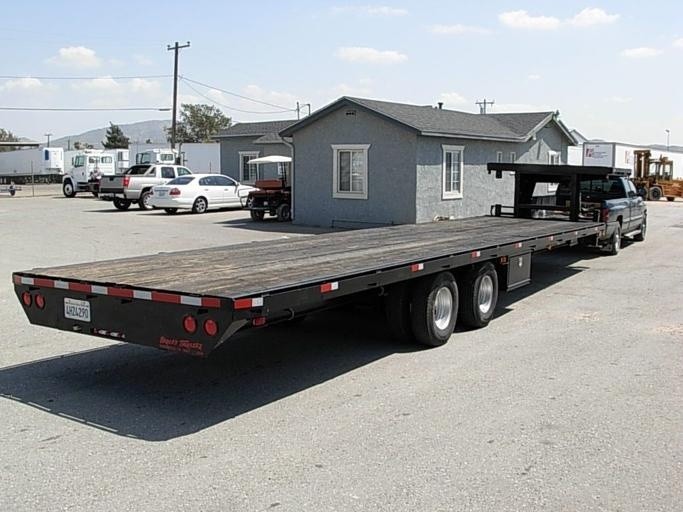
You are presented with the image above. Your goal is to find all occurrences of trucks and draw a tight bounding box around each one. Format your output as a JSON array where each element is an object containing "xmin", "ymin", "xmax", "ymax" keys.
[
  {"xmin": 137, "ymin": 149, "xmax": 176, "ymax": 166},
  {"xmin": 62, "ymin": 150, "xmax": 115, "ymax": 198}
]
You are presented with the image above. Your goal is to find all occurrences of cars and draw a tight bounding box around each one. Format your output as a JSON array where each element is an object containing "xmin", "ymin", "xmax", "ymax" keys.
[{"xmin": 146, "ymin": 173, "xmax": 256, "ymax": 214}]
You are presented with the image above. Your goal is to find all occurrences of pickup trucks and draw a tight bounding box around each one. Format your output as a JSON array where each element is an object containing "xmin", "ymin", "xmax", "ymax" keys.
[
  {"xmin": 529, "ymin": 177, "xmax": 648, "ymax": 263},
  {"xmin": 98, "ymin": 164, "xmax": 194, "ymax": 211}
]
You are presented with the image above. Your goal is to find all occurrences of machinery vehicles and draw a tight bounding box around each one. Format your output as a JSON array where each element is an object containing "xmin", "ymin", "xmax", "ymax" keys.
[{"xmin": 631, "ymin": 149, "xmax": 683, "ymax": 201}]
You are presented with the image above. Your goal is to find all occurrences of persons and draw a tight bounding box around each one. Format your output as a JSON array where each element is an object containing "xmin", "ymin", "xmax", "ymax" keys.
[{"xmin": 91, "ymin": 167, "xmax": 104, "ymax": 197}]
